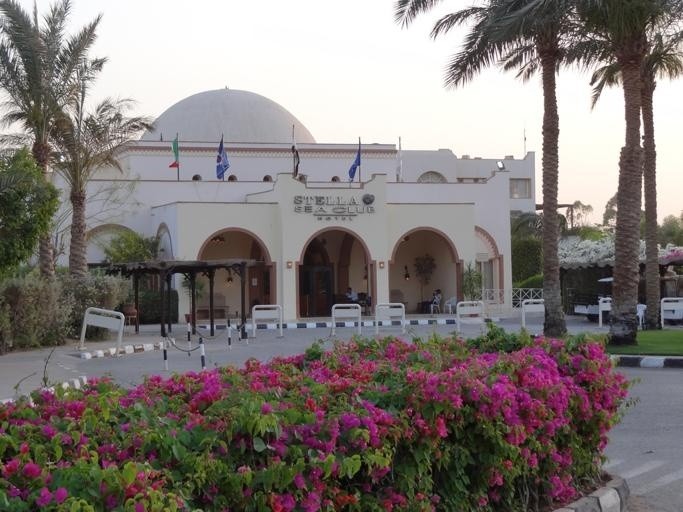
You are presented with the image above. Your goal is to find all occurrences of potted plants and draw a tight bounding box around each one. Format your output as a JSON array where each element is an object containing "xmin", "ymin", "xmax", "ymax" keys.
[
  {"xmin": 461, "ymin": 262, "xmax": 483, "ymax": 316},
  {"xmin": 182, "ymin": 276, "xmax": 204, "ymax": 323},
  {"xmin": 121, "ymin": 289, "xmax": 137, "ymax": 319}
]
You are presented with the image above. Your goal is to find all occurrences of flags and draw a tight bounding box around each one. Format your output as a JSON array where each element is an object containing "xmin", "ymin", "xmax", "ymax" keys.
[
  {"xmin": 291, "ymin": 130, "xmax": 301, "ymax": 174},
  {"xmin": 169, "ymin": 137, "xmax": 178, "ymax": 168},
  {"xmin": 216, "ymin": 138, "xmax": 230, "ymax": 179},
  {"xmin": 348, "ymin": 149, "xmax": 360, "ymax": 182}
]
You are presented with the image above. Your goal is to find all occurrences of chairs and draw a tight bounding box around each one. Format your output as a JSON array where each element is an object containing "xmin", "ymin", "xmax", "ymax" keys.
[
  {"xmin": 428, "ymin": 298, "xmax": 455, "ymax": 314},
  {"xmin": 391, "ymin": 290, "xmax": 407, "ymax": 311}
]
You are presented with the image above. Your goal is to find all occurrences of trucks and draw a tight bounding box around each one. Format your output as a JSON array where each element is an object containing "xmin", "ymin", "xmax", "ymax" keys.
[{"xmin": 568, "ymin": 287, "xmax": 608, "ymax": 321}]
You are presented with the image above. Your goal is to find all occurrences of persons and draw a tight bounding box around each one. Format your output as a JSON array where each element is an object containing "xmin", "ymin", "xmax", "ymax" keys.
[
  {"xmin": 344, "ymin": 287, "xmax": 358, "ymax": 304},
  {"xmin": 423, "ymin": 289, "xmax": 441, "ymax": 314}
]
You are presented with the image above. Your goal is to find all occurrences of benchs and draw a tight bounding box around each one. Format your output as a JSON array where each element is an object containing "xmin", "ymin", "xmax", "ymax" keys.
[{"xmin": 195, "ymin": 293, "xmax": 229, "ymax": 320}]
